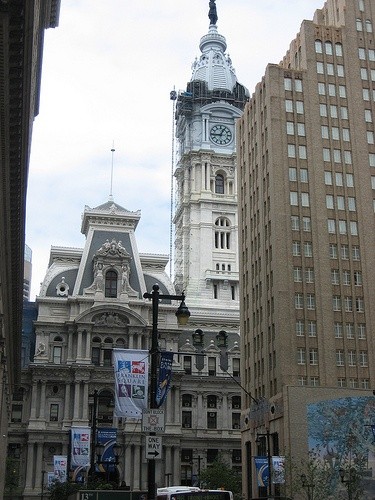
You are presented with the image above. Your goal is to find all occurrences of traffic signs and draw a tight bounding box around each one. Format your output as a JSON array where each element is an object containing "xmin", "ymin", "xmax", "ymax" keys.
[{"xmin": 145, "ymin": 435, "xmax": 163, "ymax": 460}]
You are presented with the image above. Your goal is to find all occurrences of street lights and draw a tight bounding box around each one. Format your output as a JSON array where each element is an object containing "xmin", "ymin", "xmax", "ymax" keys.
[
  {"xmin": 68, "ymin": 468, "xmax": 87, "ymax": 487},
  {"xmin": 189, "ymin": 455, "xmax": 203, "ymax": 489},
  {"xmin": 254, "ymin": 431, "xmax": 275, "ymax": 497},
  {"xmin": 337, "ymin": 468, "xmax": 356, "ymax": 500},
  {"xmin": 95, "ymin": 442, "xmax": 121, "ymax": 483},
  {"xmin": 164, "ymin": 473, "xmax": 173, "ymax": 488},
  {"xmin": 300, "ymin": 472, "xmax": 316, "ymax": 500},
  {"xmin": 142, "ymin": 283, "xmax": 191, "ymax": 500},
  {"xmin": 40, "ymin": 470, "xmax": 48, "ymax": 500},
  {"xmin": 88, "ymin": 388, "xmax": 115, "ymax": 500}
]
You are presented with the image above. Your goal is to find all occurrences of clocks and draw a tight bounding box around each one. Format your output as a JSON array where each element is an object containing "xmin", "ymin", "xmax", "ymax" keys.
[{"xmin": 210, "ymin": 124, "xmax": 231, "ymax": 144}]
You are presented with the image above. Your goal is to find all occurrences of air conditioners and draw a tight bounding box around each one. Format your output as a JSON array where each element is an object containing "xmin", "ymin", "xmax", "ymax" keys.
[
  {"xmin": 185, "ymin": 456, "xmax": 189, "ymax": 459},
  {"xmin": 108, "ymin": 416, "xmax": 113, "ymax": 420},
  {"xmin": 184, "ymin": 403, "xmax": 189, "ymax": 407},
  {"xmin": 210, "ymin": 404, "xmax": 215, "ymax": 407},
  {"xmin": 235, "ymin": 425, "xmax": 240, "ymax": 429},
  {"xmin": 210, "ymin": 413, "xmax": 215, "ymax": 416},
  {"xmin": 235, "ymin": 404, "xmax": 239, "ymax": 408},
  {"xmin": 236, "ymin": 456, "xmax": 241, "ymax": 459},
  {"xmin": 98, "ymin": 416, "xmax": 102, "ymax": 419}
]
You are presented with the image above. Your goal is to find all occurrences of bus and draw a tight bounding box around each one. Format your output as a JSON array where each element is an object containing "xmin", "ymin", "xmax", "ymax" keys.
[{"xmin": 157, "ymin": 485, "xmax": 234, "ymax": 500}]
